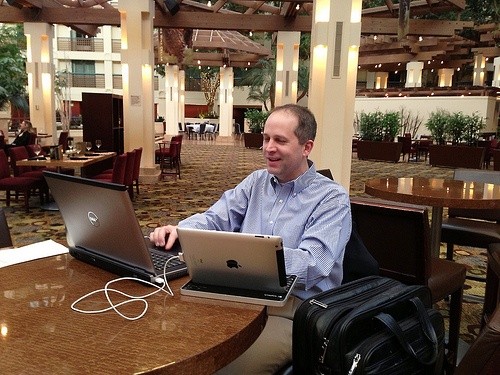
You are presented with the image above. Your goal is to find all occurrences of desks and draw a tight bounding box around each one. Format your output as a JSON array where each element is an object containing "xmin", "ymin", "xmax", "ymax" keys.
[
  {"xmin": 16, "ymin": 151, "xmax": 114, "ymax": 211},
  {"xmin": 0, "ymin": 246, "xmax": 268, "ymax": 375},
  {"xmin": 364, "ymin": 178, "xmax": 500, "ymax": 256},
  {"xmin": 186, "ymin": 124, "xmax": 214, "ymax": 140}
]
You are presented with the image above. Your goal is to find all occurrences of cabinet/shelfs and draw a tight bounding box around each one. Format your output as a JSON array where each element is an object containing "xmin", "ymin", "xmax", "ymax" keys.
[{"xmin": 82, "ymin": 93, "xmax": 124, "ymax": 154}]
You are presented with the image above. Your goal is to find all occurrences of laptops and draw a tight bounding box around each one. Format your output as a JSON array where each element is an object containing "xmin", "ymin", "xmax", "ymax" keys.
[
  {"xmin": 178, "ymin": 228, "xmax": 298, "ymax": 306},
  {"xmin": 43, "ymin": 170, "xmax": 188, "ymax": 286}
]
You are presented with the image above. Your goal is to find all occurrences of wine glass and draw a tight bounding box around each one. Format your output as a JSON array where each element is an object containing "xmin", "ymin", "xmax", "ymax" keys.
[
  {"xmin": 67, "ymin": 140, "xmax": 102, "ymax": 157},
  {"xmin": 33, "ymin": 144, "xmax": 41, "ymax": 162}
]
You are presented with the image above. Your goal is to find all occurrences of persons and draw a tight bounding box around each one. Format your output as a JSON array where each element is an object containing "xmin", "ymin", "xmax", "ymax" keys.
[
  {"xmin": 150, "ymin": 107, "xmax": 352, "ymax": 375},
  {"xmin": 9, "ymin": 120, "xmax": 35, "ymax": 147}
]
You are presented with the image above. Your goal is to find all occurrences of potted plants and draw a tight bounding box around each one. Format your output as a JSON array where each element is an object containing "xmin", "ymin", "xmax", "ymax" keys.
[
  {"xmin": 244, "ymin": 109, "xmax": 269, "ymax": 148},
  {"xmin": 357, "ymin": 111, "xmax": 401, "ymax": 161},
  {"xmin": 430, "ymin": 110, "xmax": 485, "ymax": 168}
]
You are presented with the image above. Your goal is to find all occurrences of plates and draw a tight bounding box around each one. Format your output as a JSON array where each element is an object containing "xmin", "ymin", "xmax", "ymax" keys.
[
  {"xmin": 28, "ymin": 157, "xmax": 47, "ymax": 160},
  {"xmin": 70, "ymin": 158, "xmax": 89, "ymax": 159},
  {"xmin": 85, "ymin": 154, "xmax": 99, "ymax": 156}
]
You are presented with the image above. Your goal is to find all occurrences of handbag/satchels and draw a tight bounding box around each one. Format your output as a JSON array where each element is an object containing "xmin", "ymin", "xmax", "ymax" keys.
[{"xmin": 292, "ymin": 275, "xmax": 445, "ymax": 375}]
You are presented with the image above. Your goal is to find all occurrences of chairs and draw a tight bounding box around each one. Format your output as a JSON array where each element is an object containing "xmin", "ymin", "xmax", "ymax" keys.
[
  {"xmin": 156, "ymin": 135, "xmax": 183, "ymax": 170},
  {"xmin": 59, "ymin": 132, "xmax": 69, "ymax": 150},
  {"xmin": 0, "ymin": 145, "xmax": 55, "ymax": 213},
  {"xmin": 397, "ymin": 133, "xmax": 433, "ymax": 163},
  {"xmin": 90, "ymin": 148, "xmax": 142, "ymax": 198},
  {"xmin": 188, "ymin": 123, "xmax": 216, "ymax": 141},
  {"xmin": 233, "ymin": 123, "xmax": 244, "ymax": 140},
  {"xmin": 351, "ymin": 168, "xmax": 500, "ymax": 369}
]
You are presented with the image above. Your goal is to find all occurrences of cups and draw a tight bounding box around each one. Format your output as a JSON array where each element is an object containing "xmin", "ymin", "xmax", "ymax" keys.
[{"xmin": 55, "ymin": 147, "xmax": 62, "ymax": 160}]
